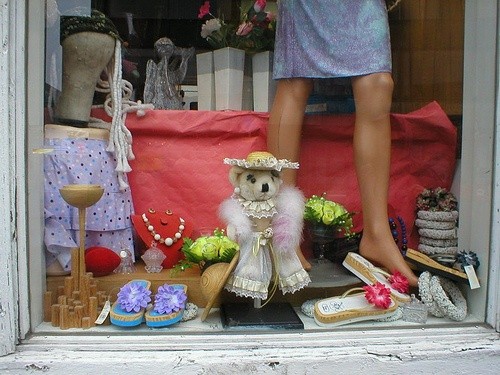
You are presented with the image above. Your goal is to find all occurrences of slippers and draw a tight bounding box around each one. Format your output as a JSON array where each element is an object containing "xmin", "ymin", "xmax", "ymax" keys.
[
  {"xmin": 145, "ymin": 283, "xmax": 189, "ymax": 327},
  {"xmin": 109, "ymin": 279, "xmax": 151, "ymax": 327},
  {"xmin": 314, "ymin": 283, "xmax": 398, "ymax": 327},
  {"xmin": 406, "ymin": 248, "xmax": 480, "ymax": 283},
  {"xmin": 343, "ymin": 252, "xmax": 411, "ymax": 305}
]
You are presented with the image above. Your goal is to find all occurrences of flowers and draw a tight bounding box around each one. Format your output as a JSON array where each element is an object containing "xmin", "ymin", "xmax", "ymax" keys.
[
  {"xmin": 362, "ymin": 280, "xmax": 391, "ymax": 309},
  {"xmin": 185, "ymin": 231, "xmax": 240, "ymax": 263},
  {"xmin": 199, "ymin": 0, "xmax": 267, "ymax": 47},
  {"xmin": 455, "ymin": 249, "xmax": 480, "ymax": 270},
  {"xmin": 117, "ymin": 282, "xmax": 152, "ymax": 312},
  {"xmin": 415, "ymin": 187, "xmax": 458, "ymax": 213},
  {"xmin": 304, "ymin": 192, "xmax": 356, "ymax": 240},
  {"xmin": 389, "ymin": 270, "xmax": 409, "ymax": 293},
  {"xmin": 154, "ymin": 283, "xmax": 188, "ymax": 314}
]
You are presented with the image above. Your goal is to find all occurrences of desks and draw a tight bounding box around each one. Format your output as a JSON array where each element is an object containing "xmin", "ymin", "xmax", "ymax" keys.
[{"xmin": 46, "ymin": 101, "xmax": 458, "ymax": 265}]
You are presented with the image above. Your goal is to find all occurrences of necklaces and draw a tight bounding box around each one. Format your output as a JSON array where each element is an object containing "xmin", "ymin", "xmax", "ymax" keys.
[
  {"xmin": 142, "ymin": 213, "xmax": 184, "ymax": 246},
  {"xmin": 388, "ymin": 217, "xmax": 407, "ymax": 259}
]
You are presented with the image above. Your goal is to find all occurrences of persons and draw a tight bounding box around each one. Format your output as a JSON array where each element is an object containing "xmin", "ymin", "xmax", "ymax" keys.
[{"xmin": 267, "ymin": 0, "xmax": 420, "ymax": 288}]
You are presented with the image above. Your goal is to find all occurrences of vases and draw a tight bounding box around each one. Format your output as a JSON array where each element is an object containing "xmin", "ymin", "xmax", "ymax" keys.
[
  {"xmin": 311, "ymin": 224, "xmax": 336, "ymax": 265},
  {"xmin": 252, "ymin": 50, "xmax": 273, "ymax": 112},
  {"xmin": 213, "ymin": 47, "xmax": 246, "ymax": 112},
  {"xmin": 196, "ymin": 52, "xmax": 214, "ymax": 110}
]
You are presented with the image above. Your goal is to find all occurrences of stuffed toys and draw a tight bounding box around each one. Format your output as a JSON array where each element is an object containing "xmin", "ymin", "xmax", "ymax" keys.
[{"xmin": 218, "ymin": 151, "xmax": 311, "ymax": 300}]
